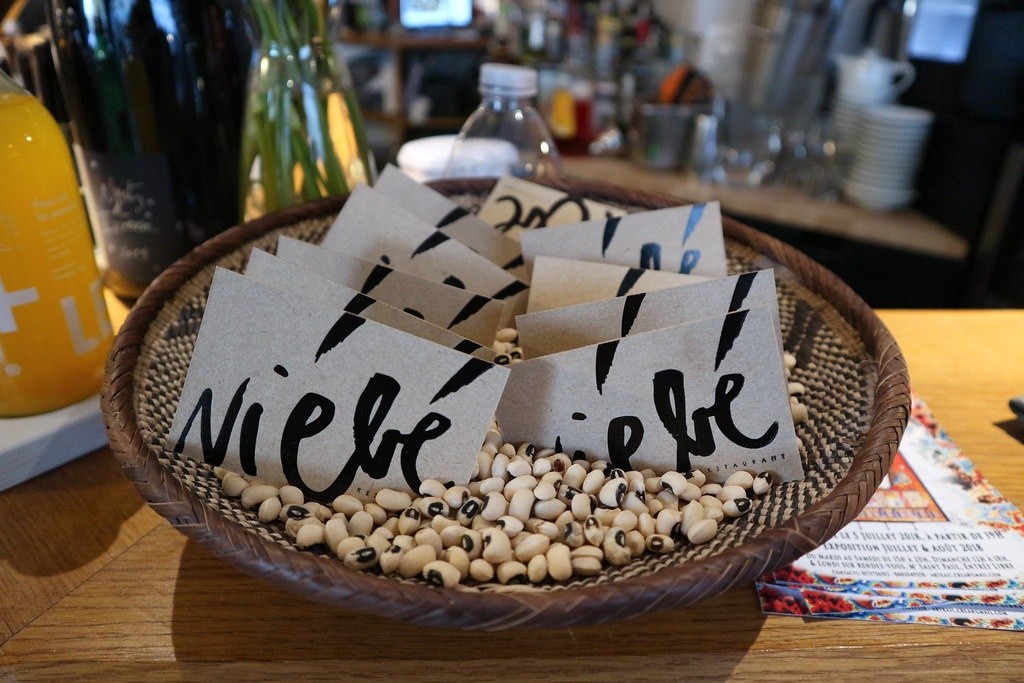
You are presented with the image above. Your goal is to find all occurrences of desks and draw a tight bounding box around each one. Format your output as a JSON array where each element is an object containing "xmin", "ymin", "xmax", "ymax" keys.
[{"xmin": 561, "ymin": 153, "xmax": 969, "ymax": 308}]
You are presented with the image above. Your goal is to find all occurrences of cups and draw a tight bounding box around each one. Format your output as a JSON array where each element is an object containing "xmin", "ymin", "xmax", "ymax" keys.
[{"xmin": 398, "ymin": 136, "xmax": 520, "ymax": 183}]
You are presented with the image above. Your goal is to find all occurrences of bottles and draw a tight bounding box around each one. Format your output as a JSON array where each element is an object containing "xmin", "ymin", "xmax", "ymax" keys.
[
  {"xmin": 0, "ymin": 70, "xmax": 115, "ymax": 417},
  {"xmin": 442, "ymin": 64, "xmax": 572, "ymax": 193}
]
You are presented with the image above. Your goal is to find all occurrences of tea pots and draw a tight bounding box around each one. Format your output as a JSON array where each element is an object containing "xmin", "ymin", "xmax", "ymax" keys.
[{"xmin": 827, "ymin": 49, "xmax": 915, "ymax": 105}]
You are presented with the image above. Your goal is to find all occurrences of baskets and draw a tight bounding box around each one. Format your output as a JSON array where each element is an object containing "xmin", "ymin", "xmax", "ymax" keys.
[{"xmin": 102, "ymin": 176, "xmax": 916, "ymax": 637}]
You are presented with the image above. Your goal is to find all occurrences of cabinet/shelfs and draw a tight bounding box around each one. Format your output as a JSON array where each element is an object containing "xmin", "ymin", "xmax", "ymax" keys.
[{"xmin": 338, "ymin": 27, "xmax": 485, "ymax": 169}]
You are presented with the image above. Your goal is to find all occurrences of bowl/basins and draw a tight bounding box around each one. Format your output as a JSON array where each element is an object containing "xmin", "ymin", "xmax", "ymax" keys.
[{"xmin": 831, "ymin": 98, "xmax": 933, "ymax": 214}]
